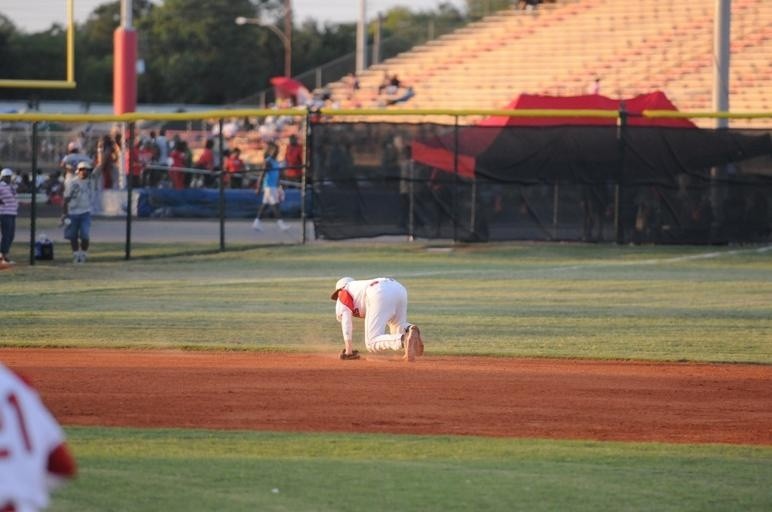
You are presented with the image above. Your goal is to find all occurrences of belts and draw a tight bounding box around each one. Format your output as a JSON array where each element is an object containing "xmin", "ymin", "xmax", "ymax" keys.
[{"xmin": 370, "ymin": 278, "xmax": 394, "ymax": 287}]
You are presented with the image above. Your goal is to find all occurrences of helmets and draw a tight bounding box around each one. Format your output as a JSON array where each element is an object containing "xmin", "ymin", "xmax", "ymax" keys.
[{"xmin": 68, "ymin": 142, "xmax": 80, "ymax": 153}]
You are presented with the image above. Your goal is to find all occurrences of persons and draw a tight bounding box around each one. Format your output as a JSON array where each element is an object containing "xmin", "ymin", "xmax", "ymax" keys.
[
  {"xmin": 62, "ymin": 135, "xmax": 107, "ymax": 263},
  {"xmin": 0, "ymin": 168, "xmax": 20, "ymax": 264},
  {"xmin": 518, "ymin": 0, "xmax": 542, "ymax": 10},
  {"xmin": 1, "ymin": 361, "xmax": 81, "ymax": 512},
  {"xmin": 328, "ymin": 275, "xmax": 425, "ymax": 362},
  {"xmin": 252, "ymin": 139, "xmax": 293, "ymax": 232}
]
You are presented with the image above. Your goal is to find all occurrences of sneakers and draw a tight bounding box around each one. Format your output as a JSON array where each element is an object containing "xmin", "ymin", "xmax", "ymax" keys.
[
  {"xmin": 402, "ymin": 335, "xmax": 416, "ymax": 362},
  {"xmin": 407, "ymin": 324, "xmax": 424, "ymax": 357},
  {"xmin": 0, "ymin": 250, "xmax": 87, "ymax": 268}
]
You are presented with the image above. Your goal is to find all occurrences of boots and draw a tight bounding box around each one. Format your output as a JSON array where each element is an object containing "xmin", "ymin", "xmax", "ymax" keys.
[{"xmin": 251, "ymin": 217, "xmax": 293, "ymax": 234}]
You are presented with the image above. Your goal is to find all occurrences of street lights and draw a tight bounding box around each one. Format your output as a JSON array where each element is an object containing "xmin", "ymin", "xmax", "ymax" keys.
[{"xmin": 234, "ymin": 15, "xmax": 293, "ymax": 79}]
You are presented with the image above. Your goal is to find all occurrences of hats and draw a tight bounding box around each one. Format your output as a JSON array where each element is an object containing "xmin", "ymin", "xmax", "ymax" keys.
[
  {"xmin": 77, "ymin": 162, "xmax": 92, "ymax": 170},
  {"xmin": 330, "ymin": 277, "xmax": 354, "ymax": 301},
  {"xmin": 1, "ymin": 168, "xmax": 13, "ymax": 177}
]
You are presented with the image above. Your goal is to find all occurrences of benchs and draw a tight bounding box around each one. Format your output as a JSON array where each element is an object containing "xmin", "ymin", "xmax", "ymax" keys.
[
  {"xmin": 192, "ymin": 114, "xmax": 301, "ymax": 165},
  {"xmin": 309, "ymin": 2, "xmax": 771, "ymax": 129}
]
